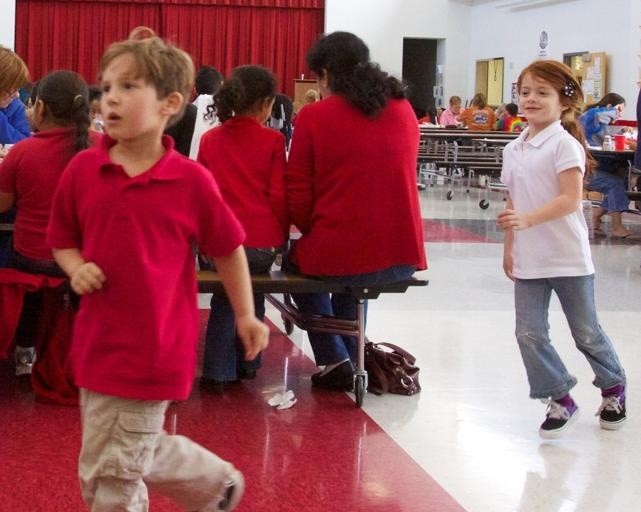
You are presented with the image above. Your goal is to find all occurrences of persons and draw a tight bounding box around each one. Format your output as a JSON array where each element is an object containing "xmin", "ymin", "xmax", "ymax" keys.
[
  {"xmin": 415, "ymin": 91, "xmax": 640, "ymax": 241},
  {"xmin": 44, "ymin": 24, "xmax": 275, "ymax": 511},
  {"xmin": 494, "ymin": 57, "xmax": 631, "ymax": 439}
]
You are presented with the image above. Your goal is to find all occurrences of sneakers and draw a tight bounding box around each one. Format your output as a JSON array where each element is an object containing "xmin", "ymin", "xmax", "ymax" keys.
[
  {"xmin": 14, "ymin": 345, "xmax": 37, "ymax": 380},
  {"xmin": 598, "ymin": 388, "xmax": 631, "ymax": 430},
  {"xmin": 536, "ymin": 393, "xmax": 580, "ymax": 439},
  {"xmin": 199, "ymin": 469, "xmax": 245, "ymax": 512},
  {"xmin": 199, "ymin": 356, "xmax": 260, "ymax": 393}
]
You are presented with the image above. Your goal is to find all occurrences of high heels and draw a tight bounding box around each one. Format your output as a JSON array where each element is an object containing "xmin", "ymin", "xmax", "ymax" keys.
[{"xmin": 311, "ymin": 358, "xmax": 355, "ymax": 392}]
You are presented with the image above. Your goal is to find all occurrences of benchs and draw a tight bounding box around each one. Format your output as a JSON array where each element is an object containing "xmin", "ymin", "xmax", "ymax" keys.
[
  {"xmin": 183, "ymin": 273, "xmax": 429, "ymax": 404},
  {"xmin": 409, "ymin": 117, "xmax": 638, "ymax": 239}
]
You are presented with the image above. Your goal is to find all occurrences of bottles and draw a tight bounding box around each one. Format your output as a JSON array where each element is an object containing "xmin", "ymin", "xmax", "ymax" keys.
[
  {"xmin": 92, "ymin": 114, "xmax": 104, "ymax": 132},
  {"xmin": 604, "ymin": 136, "xmax": 616, "ymax": 151},
  {"xmin": 302, "ymin": 74, "xmax": 305, "ymax": 80},
  {"xmin": 281, "ymin": 121, "xmax": 294, "ymax": 151}
]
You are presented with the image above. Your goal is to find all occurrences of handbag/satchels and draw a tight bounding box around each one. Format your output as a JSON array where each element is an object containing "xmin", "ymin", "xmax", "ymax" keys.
[{"xmin": 362, "ymin": 336, "xmax": 422, "ymax": 395}]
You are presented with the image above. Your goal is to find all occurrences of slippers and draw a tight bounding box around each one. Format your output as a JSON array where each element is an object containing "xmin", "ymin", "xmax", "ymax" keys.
[
  {"xmin": 611, "ymin": 231, "xmax": 640, "ymax": 242},
  {"xmin": 589, "ymin": 228, "xmax": 606, "ymax": 239}
]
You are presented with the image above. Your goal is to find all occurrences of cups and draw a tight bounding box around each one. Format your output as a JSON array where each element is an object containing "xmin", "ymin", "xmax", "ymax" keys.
[{"xmin": 615, "ymin": 135, "xmax": 625, "ymax": 151}]
